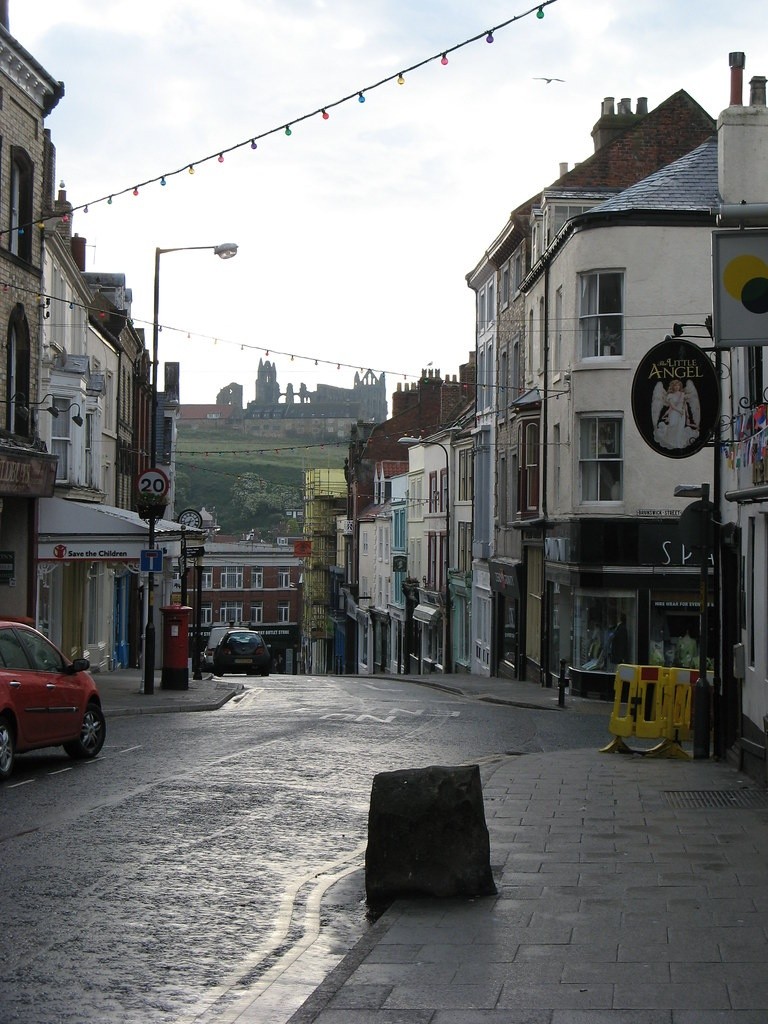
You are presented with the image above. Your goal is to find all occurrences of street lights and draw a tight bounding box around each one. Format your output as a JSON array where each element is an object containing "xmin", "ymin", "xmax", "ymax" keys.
[
  {"xmin": 144, "ymin": 242, "xmax": 240, "ymax": 695},
  {"xmin": 399, "ymin": 437, "xmax": 452, "ymax": 675}
]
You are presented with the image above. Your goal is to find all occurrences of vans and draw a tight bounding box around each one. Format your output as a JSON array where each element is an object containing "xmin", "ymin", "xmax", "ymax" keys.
[{"xmin": 204, "ymin": 626, "xmax": 249, "ymax": 664}]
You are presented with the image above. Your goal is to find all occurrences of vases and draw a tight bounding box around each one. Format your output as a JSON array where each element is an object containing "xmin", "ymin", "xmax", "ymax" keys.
[{"xmin": 134, "ymin": 504, "xmax": 168, "ymax": 518}]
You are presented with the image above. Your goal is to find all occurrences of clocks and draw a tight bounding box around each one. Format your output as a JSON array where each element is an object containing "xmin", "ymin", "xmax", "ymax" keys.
[{"xmin": 178, "ymin": 509, "xmax": 202, "ymax": 527}]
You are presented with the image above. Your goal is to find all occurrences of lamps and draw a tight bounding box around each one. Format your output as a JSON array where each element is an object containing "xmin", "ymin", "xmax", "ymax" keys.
[{"xmin": 0, "ymin": 393, "xmax": 83, "ymax": 427}]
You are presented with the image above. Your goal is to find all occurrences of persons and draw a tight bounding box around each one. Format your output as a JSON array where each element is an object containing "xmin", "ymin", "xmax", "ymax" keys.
[
  {"xmin": 274, "ymin": 654, "xmax": 284, "ymax": 674},
  {"xmin": 653, "ymin": 381, "xmax": 699, "ymax": 449}
]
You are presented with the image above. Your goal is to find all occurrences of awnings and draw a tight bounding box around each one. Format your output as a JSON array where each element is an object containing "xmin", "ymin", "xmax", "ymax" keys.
[
  {"xmin": 413, "ymin": 604, "xmax": 443, "ymax": 627},
  {"xmin": 41, "ymin": 496, "xmax": 205, "ymax": 558}
]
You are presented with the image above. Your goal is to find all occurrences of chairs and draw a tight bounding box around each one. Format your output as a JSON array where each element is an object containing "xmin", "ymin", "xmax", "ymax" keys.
[
  {"xmin": 229, "ymin": 638, "xmax": 236, "ymax": 643},
  {"xmin": 249, "ymin": 638, "xmax": 257, "ymax": 643}
]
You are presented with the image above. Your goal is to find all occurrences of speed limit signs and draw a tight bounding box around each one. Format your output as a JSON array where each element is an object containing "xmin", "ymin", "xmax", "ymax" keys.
[{"xmin": 137, "ymin": 469, "xmax": 169, "ymax": 497}]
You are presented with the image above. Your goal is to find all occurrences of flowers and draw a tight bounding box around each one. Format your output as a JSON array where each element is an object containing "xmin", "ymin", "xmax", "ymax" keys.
[{"xmin": 136, "ymin": 492, "xmax": 167, "ymax": 504}]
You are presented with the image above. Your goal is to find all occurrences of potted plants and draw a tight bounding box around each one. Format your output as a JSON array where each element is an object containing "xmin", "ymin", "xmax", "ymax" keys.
[{"xmin": 599, "ymin": 326, "xmax": 620, "ymax": 356}]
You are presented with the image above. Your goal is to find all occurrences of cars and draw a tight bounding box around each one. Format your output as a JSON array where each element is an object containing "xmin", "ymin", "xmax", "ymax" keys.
[
  {"xmin": 211, "ymin": 630, "xmax": 272, "ymax": 677},
  {"xmin": 0, "ymin": 621, "xmax": 106, "ymax": 783}
]
